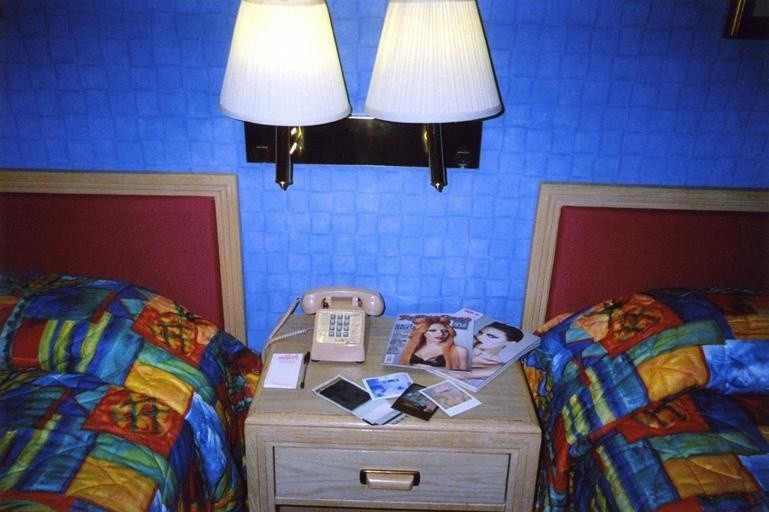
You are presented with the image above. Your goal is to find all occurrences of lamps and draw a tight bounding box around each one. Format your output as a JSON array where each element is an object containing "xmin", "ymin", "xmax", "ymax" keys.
[{"xmin": 221, "ymin": 0, "xmax": 503, "ymax": 193}]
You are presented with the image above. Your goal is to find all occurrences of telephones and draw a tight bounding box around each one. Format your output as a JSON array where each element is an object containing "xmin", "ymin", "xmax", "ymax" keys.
[{"xmin": 302, "ymin": 287, "xmax": 385, "ymax": 362}]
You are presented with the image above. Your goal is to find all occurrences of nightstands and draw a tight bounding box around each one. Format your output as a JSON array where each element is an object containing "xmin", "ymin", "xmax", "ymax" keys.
[{"xmin": 241, "ymin": 313, "xmax": 542, "ymax": 512}]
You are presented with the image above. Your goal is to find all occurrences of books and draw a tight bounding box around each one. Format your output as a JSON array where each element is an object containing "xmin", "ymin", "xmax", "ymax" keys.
[
  {"xmin": 381, "ymin": 313, "xmax": 474, "ymax": 373},
  {"xmin": 263, "ymin": 353, "xmax": 303, "ymax": 390},
  {"xmin": 423, "ymin": 307, "xmax": 542, "ymax": 393}
]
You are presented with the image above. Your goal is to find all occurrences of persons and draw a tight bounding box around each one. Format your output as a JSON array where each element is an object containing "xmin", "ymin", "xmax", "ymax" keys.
[
  {"xmin": 453, "ymin": 320, "xmax": 524, "ymax": 379},
  {"xmin": 403, "ymin": 315, "xmax": 468, "ymax": 371}
]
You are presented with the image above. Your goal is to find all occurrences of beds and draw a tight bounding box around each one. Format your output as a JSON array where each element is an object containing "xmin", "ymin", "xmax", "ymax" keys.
[
  {"xmin": 521, "ymin": 183, "xmax": 768, "ymax": 510},
  {"xmin": 0, "ymin": 169, "xmax": 249, "ymax": 511}
]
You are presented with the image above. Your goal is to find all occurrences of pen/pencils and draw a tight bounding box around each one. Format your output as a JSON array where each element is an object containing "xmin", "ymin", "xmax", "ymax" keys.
[{"xmin": 300, "ymin": 351, "xmax": 310, "ymax": 388}]
